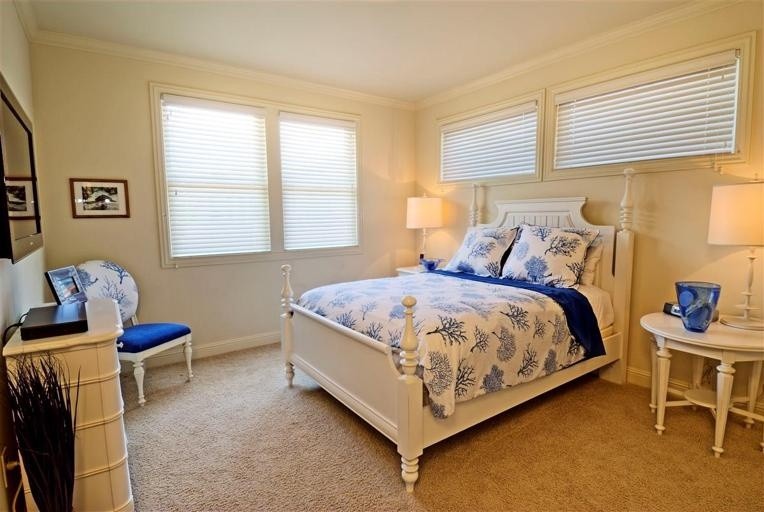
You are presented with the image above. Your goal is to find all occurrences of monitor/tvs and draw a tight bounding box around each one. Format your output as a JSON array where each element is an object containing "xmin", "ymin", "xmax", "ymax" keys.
[{"xmin": 1, "ymin": 70, "xmax": 46, "ymax": 265}]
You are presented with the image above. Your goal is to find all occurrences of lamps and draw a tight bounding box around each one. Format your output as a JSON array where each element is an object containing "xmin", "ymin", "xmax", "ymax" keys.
[
  {"xmin": 705, "ymin": 182, "xmax": 764, "ymax": 331},
  {"xmin": 405, "ymin": 191, "xmax": 443, "ymax": 265}
]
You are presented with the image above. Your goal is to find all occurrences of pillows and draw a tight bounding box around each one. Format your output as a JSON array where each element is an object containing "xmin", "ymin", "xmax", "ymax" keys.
[
  {"xmin": 442, "ymin": 225, "xmax": 520, "ymax": 279},
  {"xmin": 498, "ymin": 219, "xmax": 599, "ymax": 291}
]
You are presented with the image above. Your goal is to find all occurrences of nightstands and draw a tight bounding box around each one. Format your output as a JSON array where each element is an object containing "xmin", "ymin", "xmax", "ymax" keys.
[
  {"xmin": 638, "ymin": 306, "xmax": 760, "ymax": 460},
  {"xmin": 395, "ymin": 264, "xmax": 441, "ymax": 276}
]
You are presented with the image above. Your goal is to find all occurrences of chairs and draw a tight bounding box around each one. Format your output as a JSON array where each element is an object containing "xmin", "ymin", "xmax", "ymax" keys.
[{"xmin": 69, "ymin": 259, "xmax": 195, "ymax": 408}]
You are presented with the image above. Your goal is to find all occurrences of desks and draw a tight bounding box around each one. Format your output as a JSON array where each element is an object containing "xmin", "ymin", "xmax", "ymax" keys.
[{"xmin": 1, "ymin": 297, "xmax": 136, "ymax": 511}]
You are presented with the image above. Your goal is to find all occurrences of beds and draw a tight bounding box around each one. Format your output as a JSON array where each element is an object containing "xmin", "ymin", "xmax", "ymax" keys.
[{"xmin": 280, "ymin": 167, "xmax": 635, "ymax": 494}]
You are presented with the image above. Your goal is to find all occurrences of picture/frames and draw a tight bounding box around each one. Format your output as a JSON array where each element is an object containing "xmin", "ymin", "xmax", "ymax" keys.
[{"xmin": 69, "ymin": 177, "xmax": 131, "ymax": 219}]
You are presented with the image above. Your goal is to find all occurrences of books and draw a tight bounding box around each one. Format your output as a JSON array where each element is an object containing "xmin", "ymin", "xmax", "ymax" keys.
[{"xmin": 663, "ymin": 299, "xmax": 720, "ymax": 323}]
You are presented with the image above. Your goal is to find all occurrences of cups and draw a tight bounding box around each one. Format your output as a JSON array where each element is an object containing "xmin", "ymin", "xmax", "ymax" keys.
[
  {"xmin": 420, "ymin": 258, "xmax": 440, "ymax": 271},
  {"xmin": 675, "ymin": 281, "xmax": 721, "ymax": 333}
]
